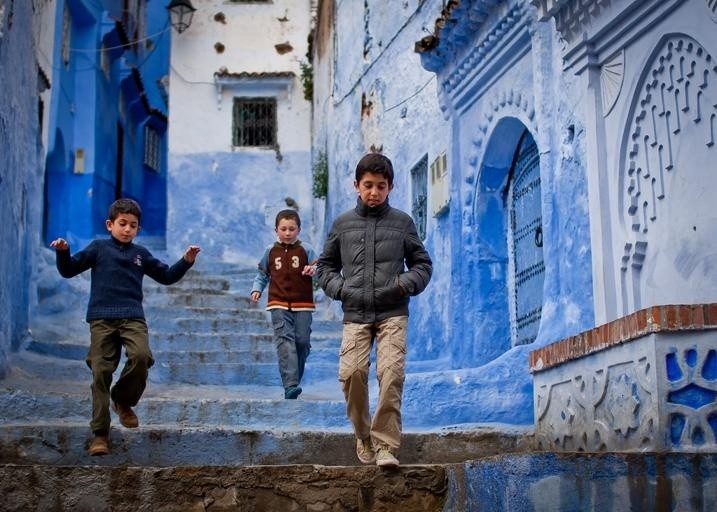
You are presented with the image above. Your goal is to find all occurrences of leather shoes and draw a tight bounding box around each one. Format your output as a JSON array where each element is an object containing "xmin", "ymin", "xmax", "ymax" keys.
[
  {"xmin": 88, "ymin": 430, "xmax": 109, "ymax": 456},
  {"xmin": 112, "ymin": 403, "xmax": 138, "ymax": 428}
]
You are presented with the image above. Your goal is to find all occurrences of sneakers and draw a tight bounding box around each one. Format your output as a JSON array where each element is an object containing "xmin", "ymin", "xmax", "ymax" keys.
[
  {"xmin": 375, "ymin": 448, "xmax": 399, "ymax": 467},
  {"xmin": 356, "ymin": 436, "xmax": 376, "ymax": 464},
  {"xmin": 284, "ymin": 384, "xmax": 302, "ymax": 399}
]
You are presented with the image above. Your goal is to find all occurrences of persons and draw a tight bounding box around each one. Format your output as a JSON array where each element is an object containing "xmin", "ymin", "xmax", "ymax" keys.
[
  {"xmin": 313, "ymin": 152, "xmax": 433, "ymax": 470},
  {"xmin": 248, "ymin": 209, "xmax": 321, "ymax": 399},
  {"xmin": 47, "ymin": 198, "xmax": 201, "ymax": 456}
]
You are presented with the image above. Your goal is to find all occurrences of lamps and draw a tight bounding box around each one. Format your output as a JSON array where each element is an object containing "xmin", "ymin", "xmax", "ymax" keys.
[{"xmin": 165, "ymin": 1, "xmax": 196, "ymax": 34}]
